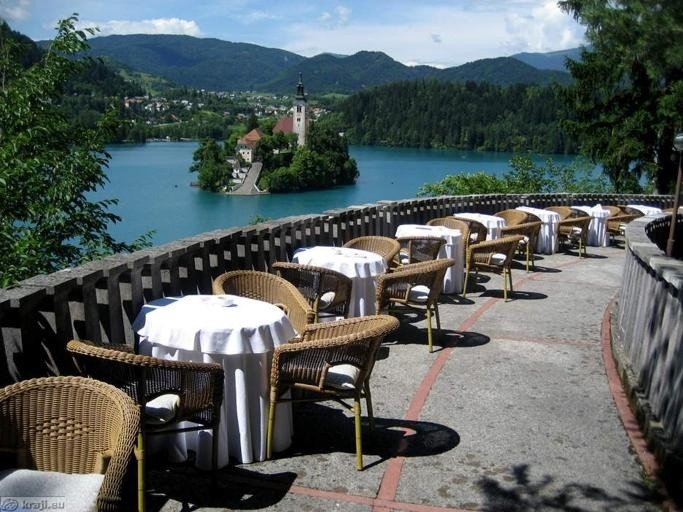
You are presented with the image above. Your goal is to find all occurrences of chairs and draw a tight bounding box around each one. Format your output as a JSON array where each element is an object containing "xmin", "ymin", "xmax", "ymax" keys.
[
  {"xmin": 374, "ymin": 258, "xmax": 454, "ymax": 352},
  {"xmin": 272, "ymin": 262, "xmax": 350, "ymax": 321},
  {"xmin": 1, "ymin": 376, "xmax": 142, "ymax": 511},
  {"xmin": 211, "ymin": 270, "xmax": 315, "ymax": 335},
  {"xmin": 267, "ymin": 315, "xmax": 400, "ymax": 471},
  {"xmin": 395, "ymin": 194, "xmax": 662, "ymax": 301},
  {"xmin": 65, "ymin": 340, "xmax": 227, "ymax": 512},
  {"xmin": 342, "ymin": 236, "xmax": 400, "ymax": 272}
]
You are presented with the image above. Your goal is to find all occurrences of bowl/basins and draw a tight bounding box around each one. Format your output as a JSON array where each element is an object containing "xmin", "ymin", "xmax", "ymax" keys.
[{"xmin": 218, "ymin": 296, "xmax": 234, "ymax": 306}]
